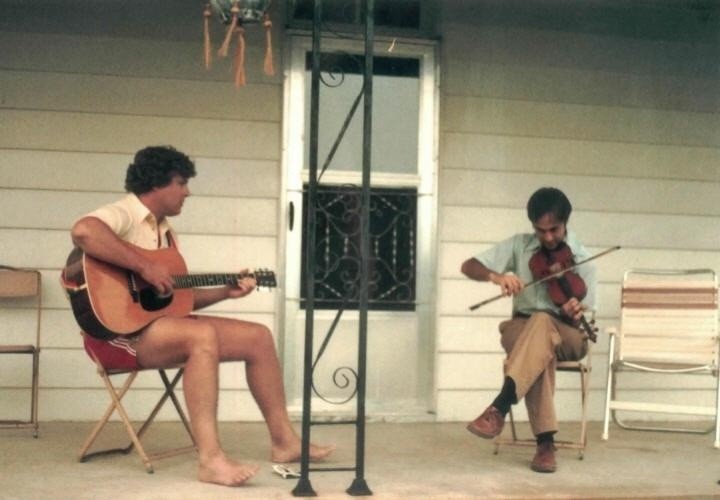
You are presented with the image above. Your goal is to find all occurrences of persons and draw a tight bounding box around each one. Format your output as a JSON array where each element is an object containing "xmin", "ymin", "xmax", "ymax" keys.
[
  {"xmin": 461, "ymin": 186, "xmax": 596, "ymax": 473},
  {"xmin": 70, "ymin": 144, "xmax": 336, "ymax": 487}
]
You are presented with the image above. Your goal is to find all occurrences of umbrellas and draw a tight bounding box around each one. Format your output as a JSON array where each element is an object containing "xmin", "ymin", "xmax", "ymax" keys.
[
  {"xmin": 466, "ymin": 405, "xmax": 504, "ymax": 440},
  {"xmin": 530, "ymin": 441, "xmax": 558, "ymax": 472}
]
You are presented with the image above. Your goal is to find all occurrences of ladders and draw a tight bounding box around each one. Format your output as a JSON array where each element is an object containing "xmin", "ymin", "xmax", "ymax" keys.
[{"xmin": 529, "ymin": 241, "xmax": 599, "ymax": 343}]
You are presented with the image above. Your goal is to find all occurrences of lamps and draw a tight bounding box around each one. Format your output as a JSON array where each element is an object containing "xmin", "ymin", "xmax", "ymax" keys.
[
  {"xmin": 60, "ymin": 277, "xmax": 200, "ymax": 474},
  {"xmin": 0, "ymin": 265, "xmax": 41, "ymax": 438},
  {"xmin": 493, "ymin": 309, "xmax": 596, "ymax": 460},
  {"xmin": 601, "ymin": 268, "xmax": 716, "ymax": 446}
]
